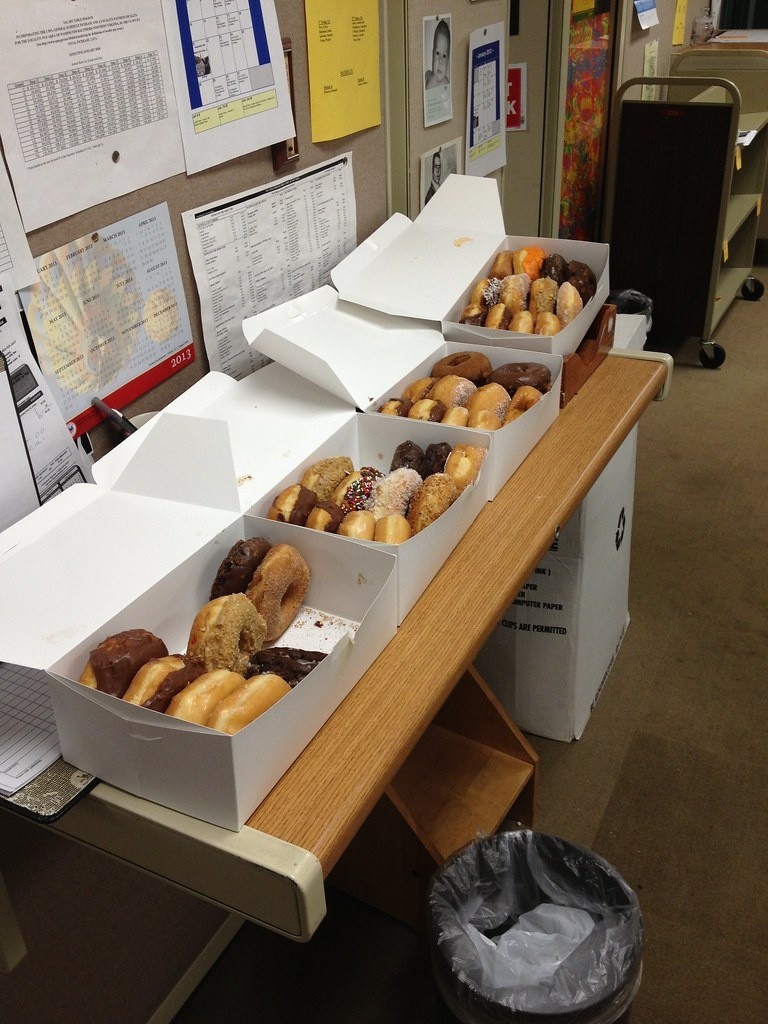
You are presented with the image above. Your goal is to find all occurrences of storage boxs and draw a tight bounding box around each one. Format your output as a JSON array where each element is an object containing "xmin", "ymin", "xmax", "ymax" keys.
[
  {"xmin": 0, "ymin": 413, "xmax": 398, "ymax": 834},
  {"xmin": 472, "ymin": 420, "xmax": 639, "ymax": 743},
  {"xmin": 93, "ymin": 362, "xmax": 493, "ymax": 628},
  {"xmin": 331, "ymin": 173, "xmax": 609, "ymax": 358},
  {"xmin": 241, "ymin": 285, "xmax": 564, "ymax": 501}
]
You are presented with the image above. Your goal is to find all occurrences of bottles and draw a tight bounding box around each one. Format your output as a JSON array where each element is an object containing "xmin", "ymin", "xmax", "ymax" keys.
[{"xmin": 691, "ymin": 7, "xmax": 713, "ymax": 45}]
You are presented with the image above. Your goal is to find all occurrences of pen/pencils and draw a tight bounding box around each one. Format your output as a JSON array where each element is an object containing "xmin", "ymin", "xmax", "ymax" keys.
[{"xmin": 91, "ymin": 395, "xmax": 137, "ymax": 433}]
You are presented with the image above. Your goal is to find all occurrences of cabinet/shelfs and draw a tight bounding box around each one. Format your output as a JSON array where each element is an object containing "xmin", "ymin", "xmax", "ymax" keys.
[
  {"xmin": 667, "ymin": 29, "xmax": 768, "ymax": 241},
  {"xmin": 601, "ymin": 49, "xmax": 768, "ymax": 368}
]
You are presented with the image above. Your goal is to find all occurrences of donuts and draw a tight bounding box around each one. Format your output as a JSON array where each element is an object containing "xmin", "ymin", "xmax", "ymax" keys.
[
  {"xmin": 265, "ymin": 438, "xmax": 488, "ymax": 545},
  {"xmin": 459, "ymin": 247, "xmax": 596, "ymax": 336},
  {"xmin": 373, "ymin": 350, "xmax": 554, "ymax": 433},
  {"xmin": 75, "ymin": 535, "xmax": 336, "ymax": 734}
]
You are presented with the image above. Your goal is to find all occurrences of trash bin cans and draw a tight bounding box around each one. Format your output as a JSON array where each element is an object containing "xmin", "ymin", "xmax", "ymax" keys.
[
  {"xmin": 423, "ymin": 829, "xmax": 644, "ymax": 1024},
  {"xmin": 607, "ymin": 292, "xmax": 652, "ymax": 333}
]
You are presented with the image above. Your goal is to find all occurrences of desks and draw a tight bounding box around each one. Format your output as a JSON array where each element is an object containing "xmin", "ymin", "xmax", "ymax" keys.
[{"xmin": 0, "ymin": 348, "xmax": 674, "ymax": 942}]
